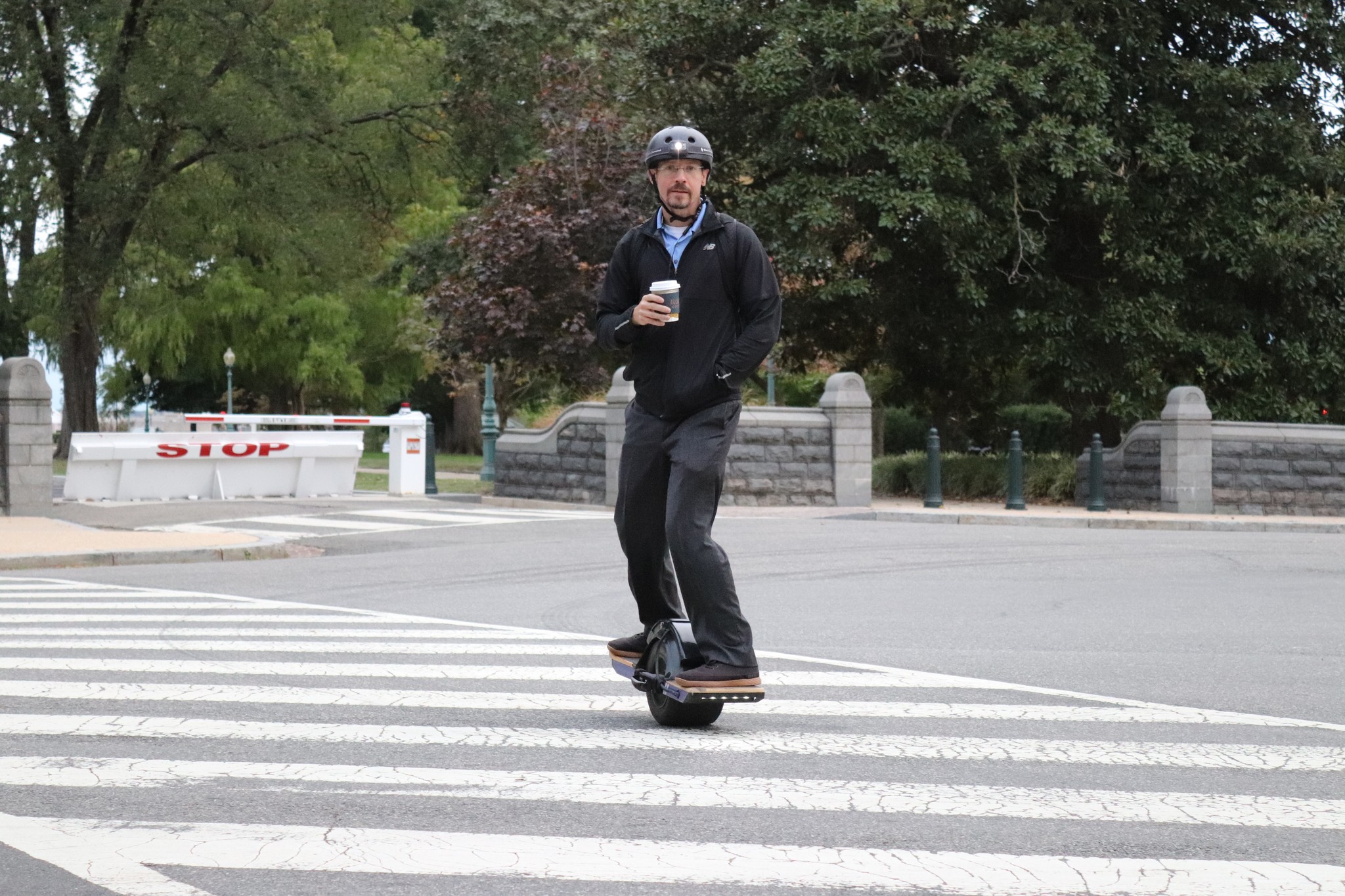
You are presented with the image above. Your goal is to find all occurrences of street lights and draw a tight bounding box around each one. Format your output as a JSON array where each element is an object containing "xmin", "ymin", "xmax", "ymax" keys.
[
  {"xmin": 223, "ymin": 346, "xmax": 236, "ymax": 431},
  {"xmin": 144, "ymin": 373, "xmax": 152, "ymax": 432}
]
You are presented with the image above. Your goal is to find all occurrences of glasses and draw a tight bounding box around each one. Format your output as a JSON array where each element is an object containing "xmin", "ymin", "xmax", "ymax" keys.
[{"xmin": 655, "ymin": 163, "xmax": 704, "ymax": 175}]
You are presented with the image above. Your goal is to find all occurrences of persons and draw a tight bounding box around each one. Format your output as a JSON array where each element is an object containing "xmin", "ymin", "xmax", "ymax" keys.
[{"xmin": 593, "ymin": 126, "xmax": 784, "ymax": 686}]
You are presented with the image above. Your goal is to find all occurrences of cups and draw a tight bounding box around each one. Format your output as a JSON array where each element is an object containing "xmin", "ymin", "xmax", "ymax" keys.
[{"xmin": 649, "ymin": 280, "xmax": 680, "ymax": 322}]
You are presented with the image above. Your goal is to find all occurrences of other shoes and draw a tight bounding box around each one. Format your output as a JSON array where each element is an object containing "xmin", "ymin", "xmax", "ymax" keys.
[
  {"xmin": 607, "ymin": 631, "xmax": 649, "ymax": 658},
  {"xmin": 674, "ymin": 659, "xmax": 762, "ymax": 688}
]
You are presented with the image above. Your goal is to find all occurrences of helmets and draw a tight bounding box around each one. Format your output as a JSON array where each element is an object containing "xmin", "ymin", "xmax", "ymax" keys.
[{"xmin": 645, "ymin": 126, "xmax": 714, "ymax": 175}]
